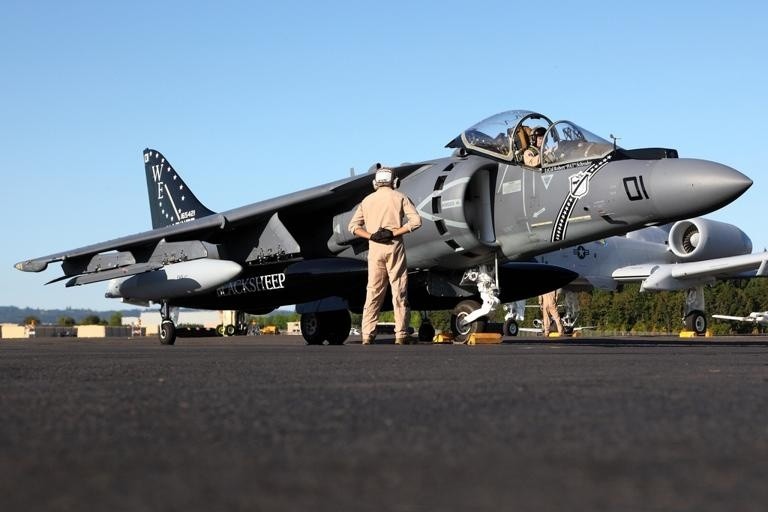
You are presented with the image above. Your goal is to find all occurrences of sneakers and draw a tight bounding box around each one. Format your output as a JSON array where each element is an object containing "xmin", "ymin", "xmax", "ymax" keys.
[
  {"xmin": 360, "ymin": 334, "xmax": 375, "ymax": 344},
  {"xmin": 394, "ymin": 335, "xmax": 418, "ymax": 345},
  {"xmin": 542, "ymin": 332, "xmax": 565, "ymax": 339}
]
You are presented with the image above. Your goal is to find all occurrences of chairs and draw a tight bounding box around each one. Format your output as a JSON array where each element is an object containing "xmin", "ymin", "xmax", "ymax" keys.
[{"xmin": 507, "ymin": 126, "xmax": 533, "ymax": 162}]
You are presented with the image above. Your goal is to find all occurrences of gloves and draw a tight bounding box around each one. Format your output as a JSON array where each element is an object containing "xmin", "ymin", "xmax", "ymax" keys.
[{"xmin": 370, "ymin": 227, "xmax": 393, "ymax": 244}]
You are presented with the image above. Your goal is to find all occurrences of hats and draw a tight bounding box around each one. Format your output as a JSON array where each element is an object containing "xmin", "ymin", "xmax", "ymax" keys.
[
  {"xmin": 529, "ymin": 128, "xmax": 547, "ymax": 146},
  {"xmin": 371, "ymin": 168, "xmax": 398, "ymax": 188}
]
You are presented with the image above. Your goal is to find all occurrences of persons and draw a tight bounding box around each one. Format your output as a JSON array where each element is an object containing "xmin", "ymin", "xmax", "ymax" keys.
[
  {"xmin": 538, "ymin": 287, "xmax": 565, "ymax": 338},
  {"xmin": 523, "ymin": 128, "xmax": 556, "ymax": 167},
  {"xmin": 347, "ymin": 166, "xmax": 423, "ymax": 345}
]
